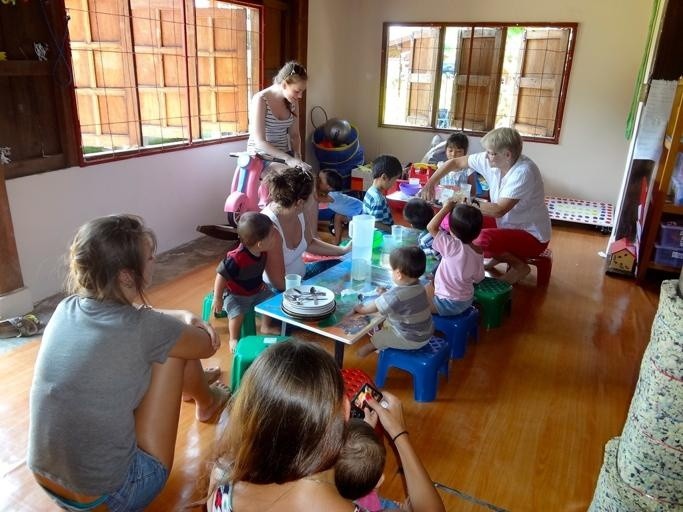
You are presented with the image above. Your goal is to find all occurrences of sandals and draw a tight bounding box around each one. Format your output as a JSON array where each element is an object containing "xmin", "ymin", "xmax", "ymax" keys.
[{"xmin": 0, "ymin": 314, "xmax": 44, "ymax": 340}]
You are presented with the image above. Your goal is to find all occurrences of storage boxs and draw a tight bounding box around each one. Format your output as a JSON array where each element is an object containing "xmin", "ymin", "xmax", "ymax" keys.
[{"xmin": 351, "ymin": 165, "xmax": 376, "ymax": 192}]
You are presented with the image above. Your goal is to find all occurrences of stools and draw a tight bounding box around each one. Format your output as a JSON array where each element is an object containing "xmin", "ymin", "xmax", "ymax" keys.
[
  {"xmin": 374, "ymin": 336, "xmax": 449, "ymax": 401},
  {"xmin": 506, "ymin": 249, "xmax": 552, "ymax": 289},
  {"xmin": 231, "ymin": 336, "xmax": 295, "ymax": 398},
  {"xmin": 431, "ymin": 306, "xmax": 479, "ymax": 360},
  {"xmin": 203, "ymin": 288, "xmax": 271, "ymax": 339},
  {"xmin": 473, "ymin": 278, "xmax": 510, "ymax": 331},
  {"xmin": 302, "ymin": 249, "xmax": 342, "ymax": 264},
  {"xmin": 339, "ymin": 370, "xmax": 383, "ymax": 441}
]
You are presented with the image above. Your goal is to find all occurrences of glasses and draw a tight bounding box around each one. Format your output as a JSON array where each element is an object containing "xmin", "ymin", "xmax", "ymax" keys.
[
  {"xmin": 288, "ymin": 65, "xmax": 306, "ymax": 74},
  {"xmin": 295, "ymin": 166, "xmax": 311, "ymax": 181}
]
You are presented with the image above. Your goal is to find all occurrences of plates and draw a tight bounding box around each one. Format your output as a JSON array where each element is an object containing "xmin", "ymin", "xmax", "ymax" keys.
[{"xmin": 281, "ymin": 285, "xmax": 339, "ymax": 321}]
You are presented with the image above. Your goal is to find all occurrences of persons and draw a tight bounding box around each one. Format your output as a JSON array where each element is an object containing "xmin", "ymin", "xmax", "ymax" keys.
[
  {"xmin": 435, "ymin": 133, "xmax": 477, "ymax": 197},
  {"xmin": 354, "ymin": 246, "xmax": 435, "ymax": 358},
  {"xmin": 29, "ymin": 214, "xmax": 230, "ymax": 512},
  {"xmin": 311, "ymin": 170, "xmax": 364, "ymax": 246},
  {"xmin": 211, "ymin": 211, "xmax": 286, "ymax": 354},
  {"xmin": 417, "ymin": 127, "xmax": 551, "ymax": 285},
  {"xmin": 259, "ymin": 169, "xmax": 354, "ymax": 294},
  {"xmin": 206, "ymin": 337, "xmax": 448, "ymax": 512},
  {"xmin": 427, "ymin": 200, "xmax": 485, "ymax": 317},
  {"xmin": 404, "ymin": 196, "xmax": 450, "ymax": 260},
  {"xmin": 362, "ymin": 155, "xmax": 402, "ymax": 224},
  {"xmin": 247, "ymin": 62, "xmax": 319, "ymax": 237},
  {"xmin": 330, "ymin": 405, "xmax": 385, "ymax": 498}
]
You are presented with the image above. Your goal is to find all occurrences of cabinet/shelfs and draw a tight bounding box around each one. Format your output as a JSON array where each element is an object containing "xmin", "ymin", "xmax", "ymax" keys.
[{"xmin": 634, "ymin": 74, "xmax": 683, "ymax": 279}]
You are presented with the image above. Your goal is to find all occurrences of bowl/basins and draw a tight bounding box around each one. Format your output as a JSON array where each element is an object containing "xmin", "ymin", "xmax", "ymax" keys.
[
  {"xmin": 439, "ymin": 189, "xmax": 455, "ymax": 206},
  {"xmin": 400, "ymin": 184, "xmax": 421, "ymax": 196}
]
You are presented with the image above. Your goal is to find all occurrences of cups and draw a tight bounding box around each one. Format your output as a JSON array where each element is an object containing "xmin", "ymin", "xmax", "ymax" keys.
[
  {"xmin": 392, "ymin": 225, "xmax": 404, "ymax": 243},
  {"xmin": 351, "ymin": 262, "xmax": 375, "ymax": 280},
  {"xmin": 348, "ymin": 214, "xmax": 376, "ymax": 260},
  {"xmin": 461, "ymin": 183, "xmax": 472, "ymax": 201},
  {"xmin": 285, "ymin": 274, "xmax": 301, "ymax": 288},
  {"xmin": 384, "ymin": 236, "xmax": 393, "ymax": 248}
]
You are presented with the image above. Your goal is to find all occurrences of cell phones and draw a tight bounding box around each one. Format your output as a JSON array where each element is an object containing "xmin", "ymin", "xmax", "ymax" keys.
[{"xmin": 350, "ymin": 382, "xmax": 381, "ymax": 418}]
